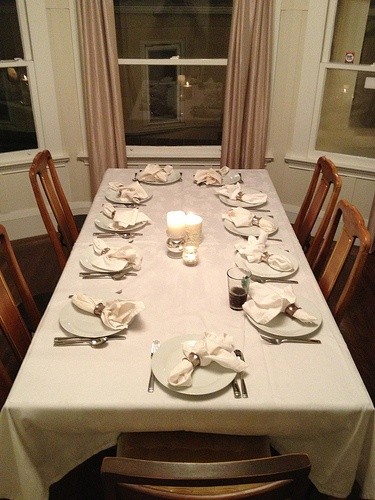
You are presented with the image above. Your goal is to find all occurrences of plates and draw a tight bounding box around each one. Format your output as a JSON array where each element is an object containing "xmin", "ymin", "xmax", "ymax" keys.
[
  {"xmin": 105, "ymin": 187, "xmax": 153, "ymax": 204},
  {"xmin": 95, "ymin": 207, "xmax": 147, "ymax": 232},
  {"xmin": 211, "ymin": 169, "xmax": 240, "ymax": 186},
  {"xmin": 152, "ymin": 334, "xmax": 238, "ymax": 395},
  {"xmin": 219, "ymin": 184, "xmax": 266, "ymax": 208},
  {"xmin": 143, "ymin": 167, "xmax": 181, "ymax": 185},
  {"xmin": 59, "ymin": 301, "xmax": 136, "ymax": 338},
  {"xmin": 247, "ymin": 294, "xmax": 322, "ymax": 338},
  {"xmin": 80, "ymin": 242, "xmax": 142, "ymax": 273},
  {"xmin": 235, "ymin": 245, "xmax": 298, "ymax": 278},
  {"xmin": 224, "ymin": 212, "xmax": 278, "ymax": 237}
]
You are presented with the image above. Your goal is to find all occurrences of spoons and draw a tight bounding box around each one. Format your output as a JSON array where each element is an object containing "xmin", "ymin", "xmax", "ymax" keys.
[
  {"xmin": 249, "ymin": 274, "xmax": 299, "ymax": 285},
  {"xmin": 54, "ymin": 337, "xmax": 108, "ymax": 345},
  {"xmin": 96, "ymin": 233, "xmax": 130, "ymax": 239},
  {"xmin": 234, "ymin": 349, "xmax": 248, "ymax": 399},
  {"xmin": 83, "ymin": 273, "xmax": 126, "ymax": 280}
]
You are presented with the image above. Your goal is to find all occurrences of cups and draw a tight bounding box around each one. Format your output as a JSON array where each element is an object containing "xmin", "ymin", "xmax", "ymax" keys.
[
  {"xmin": 165, "ymin": 210, "xmax": 187, "ymax": 252},
  {"xmin": 227, "ymin": 267, "xmax": 252, "ymax": 310},
  {"xmin": 184, "ymin": 214, "xmax": 202, "ymax": 246}
]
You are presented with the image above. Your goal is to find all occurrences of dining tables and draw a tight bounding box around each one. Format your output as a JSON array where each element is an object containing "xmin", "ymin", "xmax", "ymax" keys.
[{"xmin": 0, "ymin": 167, "xmax": 374, "ymax": 500}]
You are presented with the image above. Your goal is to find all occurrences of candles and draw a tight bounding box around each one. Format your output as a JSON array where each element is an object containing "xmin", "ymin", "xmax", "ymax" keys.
[
  {"xmin": 167, "ymin": 210, "xmax": 184, "ymax": 237},
  {"xmin": 185, "ymin": 212, "xmax": 202, "ymax": 243}
]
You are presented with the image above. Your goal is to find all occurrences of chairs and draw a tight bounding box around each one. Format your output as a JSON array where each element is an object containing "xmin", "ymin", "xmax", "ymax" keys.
[
  {"xmin": 292, "ymin": 156, "xmax": 342, "ymax": 265},
  {"xmin": 312, "ymin": 200, "xmax": 372, "ymax": 325},
  {"xmin": 0, "ymin": 224, "xmax": 42, "ymax": 402},
  {"xmin": 28, "ymin": 151, "xmax": 79, "ymax": 265},
  {"xmin": 102, "ymin": 431, "xmax": 310, "ymax": 500}
]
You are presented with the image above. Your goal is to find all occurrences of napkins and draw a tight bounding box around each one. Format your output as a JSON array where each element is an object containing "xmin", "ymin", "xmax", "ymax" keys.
[
  {"xmin": 224, "ymin": 208, "xmax": 273, "ymax": 231},
  {"xmin": 72, "ymin": 294, "xmax": 143, "ymax": 332},
  {"xmin": 91, "ymin": 236, "xmax": 143, "ymax": 275},
  {"xmin": 193, "ymin": 165, "xmax": 229, "ymax": 187},
  {"xmin": 235, "ymin": 234, "xmax": 293, "ymax": 273},
  {"xmin": 242, "ymin": 284, "xmax": 319, "ymax": 329},
  {"xmin": 107, "ymin": 180, "xmax": 145, "ymax": 205},
  {"xmin": 170, "ymin": 331, "xmax": 246, "ymax": 390},
  {"xmin": 220, "ymin": 185, "xmax": 265, "ymax": 207},
  {"xmin": 101, "ymin": 202, "xmax": 150, "ymax": 232},
  {"xmin": 138, "ymin": 162, "xmax": 172, "ymax": 182}
]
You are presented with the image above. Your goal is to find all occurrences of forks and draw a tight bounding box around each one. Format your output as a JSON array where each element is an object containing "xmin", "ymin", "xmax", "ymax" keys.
[
  {"xmin": 148, "ymin": 339, "xmax": 160, "ymax": 392},
  {"xmin": 260, "ymin": 335, "xmax": 321, "ymax": 346},
  {"xmin": 132, "ymin": 172, "xmax": 137, "ymax": 181},
  {"xmin": 68, "ymin": 289, "xmax": 122, "ymax": 298}
]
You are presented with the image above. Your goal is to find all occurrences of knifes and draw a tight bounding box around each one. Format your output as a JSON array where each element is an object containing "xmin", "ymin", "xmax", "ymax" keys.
[
  {"xmin": 93, "ymin": 232, "xmax": 144, "ymax": 237},
  {"xmin": 54, "ymin": 335, "xmax": 126, "ymax": 340},
  {"xmin": 79, "ymin": 272, "xmax": 138, "ymax": 277}
]
